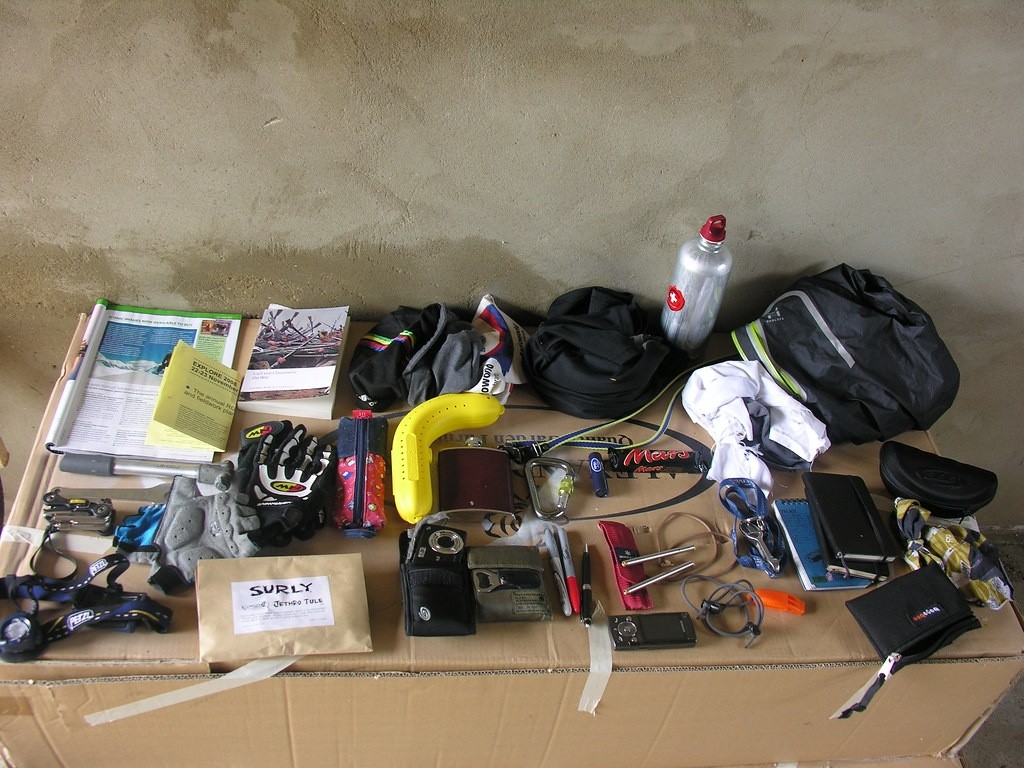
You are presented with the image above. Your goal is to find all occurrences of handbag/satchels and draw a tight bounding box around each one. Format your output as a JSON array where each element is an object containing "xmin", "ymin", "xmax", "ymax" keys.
[
  {"xmin": 738, "ymin": 267, "xmax": 962, "ymax": 444},
  {"xmin": 523, "ymin": 287, "xmax": 684, "ymax": 419}
]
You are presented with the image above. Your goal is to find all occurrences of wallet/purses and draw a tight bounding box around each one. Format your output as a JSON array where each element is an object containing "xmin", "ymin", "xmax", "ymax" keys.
[{"xmin": 845, "ymin": 561, "xmax": 982, "ymax": 674}]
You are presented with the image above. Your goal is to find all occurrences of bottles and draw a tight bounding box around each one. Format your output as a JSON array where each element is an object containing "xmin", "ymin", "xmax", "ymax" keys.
[
  {"xmin": 660, "ymin": 215, "xmax": 733, "ymax": 352},
  {"xmin": 438, "ymin": 437, "xmax": 516, "ymax": 523}
]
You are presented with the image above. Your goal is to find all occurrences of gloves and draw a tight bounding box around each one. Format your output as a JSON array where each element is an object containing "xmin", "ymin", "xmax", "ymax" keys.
[{"xmin": 239, "ymin": 422, "xmax": 338, "ymax": 547}]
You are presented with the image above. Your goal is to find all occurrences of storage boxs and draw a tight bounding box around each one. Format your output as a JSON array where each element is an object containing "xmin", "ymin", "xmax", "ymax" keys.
[{"xmin": 0, "ymin": 310, "xmax": 1024, "ymax": 768}]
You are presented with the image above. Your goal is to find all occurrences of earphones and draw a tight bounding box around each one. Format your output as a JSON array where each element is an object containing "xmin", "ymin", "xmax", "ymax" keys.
[
  {"xmin": 745, "ymin": 621, "xmax": 762, "ymax": 635},
  {"xmin": 701, "ymin": 599, "xmax": 725, "ymax": 614}
]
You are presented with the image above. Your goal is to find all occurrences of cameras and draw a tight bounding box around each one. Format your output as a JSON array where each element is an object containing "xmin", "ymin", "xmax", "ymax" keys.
[{"xmin": 412, "ymin": 523, "xmax": 468, "ymax": 562}]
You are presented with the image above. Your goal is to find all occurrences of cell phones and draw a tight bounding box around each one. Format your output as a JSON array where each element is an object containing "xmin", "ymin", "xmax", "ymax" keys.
[{"xmin": 608, "ymin": 611, "xmax": 697, "ymax": 651}]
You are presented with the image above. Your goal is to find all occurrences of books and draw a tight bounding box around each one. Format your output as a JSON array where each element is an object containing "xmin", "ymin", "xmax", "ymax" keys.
[
  {"xmin": 44, "ymin": 298, "xmax": 241, "ymax": 464},
  {"xmin": 237, "ymin": 306, "xmax": 350, "ymax": 420},
  {"xmin": 772, "ymin": 471, "xmax": 897, "ymax": 592}
]
